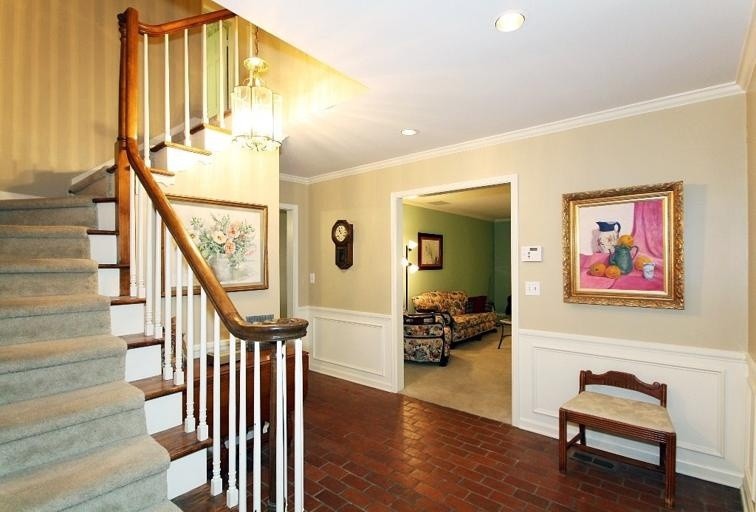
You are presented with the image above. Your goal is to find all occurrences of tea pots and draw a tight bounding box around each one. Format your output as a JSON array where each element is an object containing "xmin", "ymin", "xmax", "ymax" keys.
[
  {"xmin": 607, "ymin": 243, "xmax": 639, "ymax": 274},
  {"xmin": 596, "ymin": 220, "xmax": 622, "ymax": 255}
]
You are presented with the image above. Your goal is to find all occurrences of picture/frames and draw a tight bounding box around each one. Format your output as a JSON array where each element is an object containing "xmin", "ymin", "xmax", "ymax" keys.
[
  {"xmin": 417, "ymin": 231, "xmax": 445, "ymax": 271},
  {"xmin": 559, "ymin": 179, "xmax": 688, "ymax": 313},
  {"xmin": 155, "ymin": 190, "xmax": 272, "ymax": 297}
]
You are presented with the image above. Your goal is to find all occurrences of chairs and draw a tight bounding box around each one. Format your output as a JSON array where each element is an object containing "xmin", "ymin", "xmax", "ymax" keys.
[{"xmin": 557, "ymin": 368, "xmax": 680, "ymax": 509}]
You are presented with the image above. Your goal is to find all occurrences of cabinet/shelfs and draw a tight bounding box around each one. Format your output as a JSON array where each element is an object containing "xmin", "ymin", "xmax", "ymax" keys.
[{"xmin": 184, "ymin": 345, "xmax": 310, "ymax": 473}]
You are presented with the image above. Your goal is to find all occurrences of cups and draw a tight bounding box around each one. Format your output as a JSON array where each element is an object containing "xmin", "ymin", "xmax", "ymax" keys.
[{"xmin": 642, "ymin": 263, "xmax": 655, "ymax": 279}]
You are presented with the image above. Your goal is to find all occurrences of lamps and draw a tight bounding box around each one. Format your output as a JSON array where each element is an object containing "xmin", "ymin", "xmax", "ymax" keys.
[
  {"xmin": 229, "ymin": 21, "xmax": 286, "ymax": 154},
  {"xmin": 400, "ymin": 240, "xmax": 421, "ymax": 311},
  {"xmin": 398, "ymin": 127, "xmax": 421, "ymax": 138},
  {"xmin": 494, "ymin": 10, "xmax": 527, "ymax": 34}
]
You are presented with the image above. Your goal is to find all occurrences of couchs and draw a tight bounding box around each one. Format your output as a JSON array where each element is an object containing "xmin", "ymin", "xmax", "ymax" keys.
[{"xmin": 403, "ymin": 289, "xmax": 501, "ymax": 368}]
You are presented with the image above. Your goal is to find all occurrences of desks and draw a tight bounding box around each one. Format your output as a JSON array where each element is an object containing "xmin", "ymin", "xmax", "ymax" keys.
[{"xmin": 497, "ymin": 316, "xmax": 512, "ymax": 350}]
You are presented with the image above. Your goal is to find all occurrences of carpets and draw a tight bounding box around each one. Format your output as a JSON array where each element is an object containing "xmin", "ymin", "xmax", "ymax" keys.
[{"xmin": 0, "ymin": 126, "xmax": 184, "ymax": 512}]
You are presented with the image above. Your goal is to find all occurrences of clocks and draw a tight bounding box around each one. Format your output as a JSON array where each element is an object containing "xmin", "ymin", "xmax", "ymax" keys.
[{"xmin": 329, "ymin": 218, "xmax": 354, "ymax": 271}]
